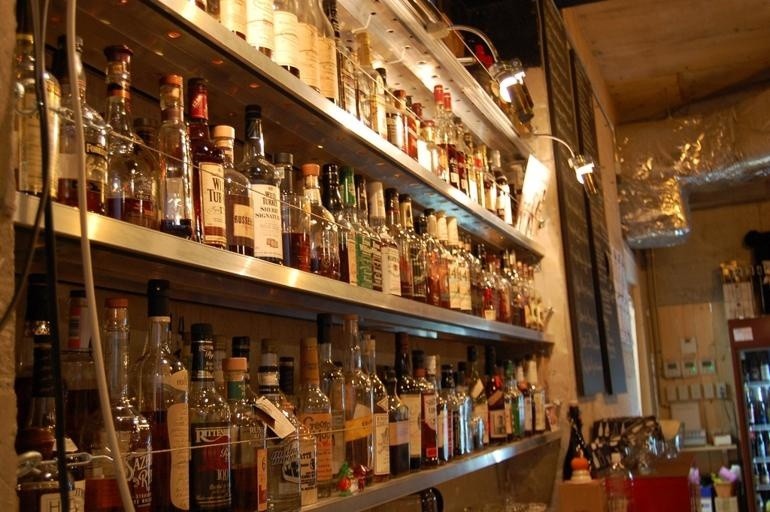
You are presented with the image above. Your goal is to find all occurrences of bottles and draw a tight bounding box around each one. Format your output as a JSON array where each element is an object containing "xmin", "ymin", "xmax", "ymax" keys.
[
  {"xmin": 19, "ymin": 279, "xmax": 546, "ymax": 512},
  {"xmin": 562, "ymin": 401, "xmax": 596, "ymax": 481},
  {"xmin": 12, "ymin": 0, "xmax": 545, "ymax": 333},
  {"xmin": 571, "ymin": 457, "xmax": 592, "ymax": 481},
  {"xmin": 604, "ymin": 452, "xmax": 633, "ymax": 511},
  {"xmin": 739, "ymin": 350, "xmax": 770, "ymax": 512}
]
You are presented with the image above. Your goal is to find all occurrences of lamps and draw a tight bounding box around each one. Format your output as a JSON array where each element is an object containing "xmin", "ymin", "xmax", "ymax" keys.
[
  {"xmin": 426, "ymin": 23, "xmax": 534, "ymax": 121},
  {"xmin": 521, "ymin": 132, "xmax": 599, "ymax": 201}
]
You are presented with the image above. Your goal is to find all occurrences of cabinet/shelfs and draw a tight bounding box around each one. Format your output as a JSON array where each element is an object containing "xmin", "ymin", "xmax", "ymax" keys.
[
  {"xmin": 0, "ymin": 1, "xmax": 562, "ymax": 512},
  {"xmin": 727, "ymin": 315, "xmax": 770, "ymax": 510}
]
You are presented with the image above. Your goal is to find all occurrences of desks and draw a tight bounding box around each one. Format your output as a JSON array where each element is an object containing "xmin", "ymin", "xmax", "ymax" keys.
[
  {"xmin": 594, "ymin": 450, "xmax": 700, "ymax": 511},
  {"xmin": 679, "ymin": 444, "xmax": 738, "ymax": 466}
]
